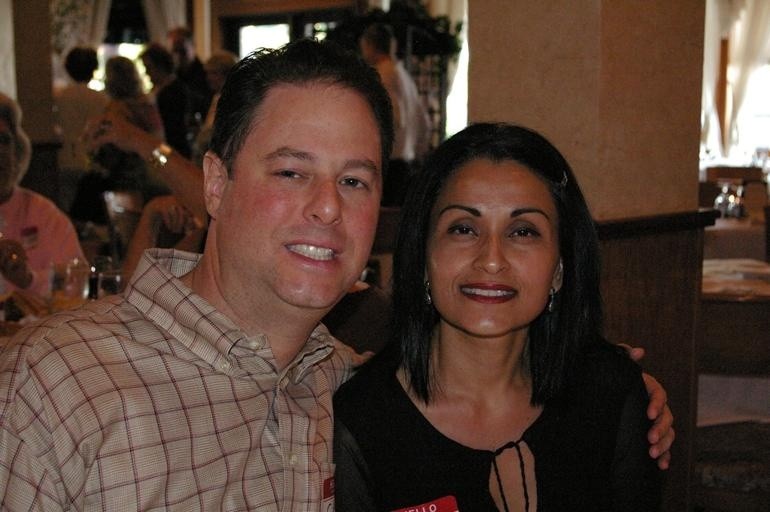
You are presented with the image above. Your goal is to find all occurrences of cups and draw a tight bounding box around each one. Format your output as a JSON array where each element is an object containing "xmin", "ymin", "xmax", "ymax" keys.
[{"xmin": 47, "ymin": 259, "xmax": 129, "ymax": 309}]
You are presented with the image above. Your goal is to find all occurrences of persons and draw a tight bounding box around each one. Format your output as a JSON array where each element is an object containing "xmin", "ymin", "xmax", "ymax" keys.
[
  {"xmin": 362, "ymin": 24, "xmax": 433, "ymax": 172},
  {"xmin": 0, "ymin": 36, "xmax": 675, "ymax": 512},
  {"xmin": 0, "ymin": 27, "xmax": 242, "ymax": 329},
  {"xmin": 333, "ymin": 120, "xmax": 667, "ymax": 512}
]
